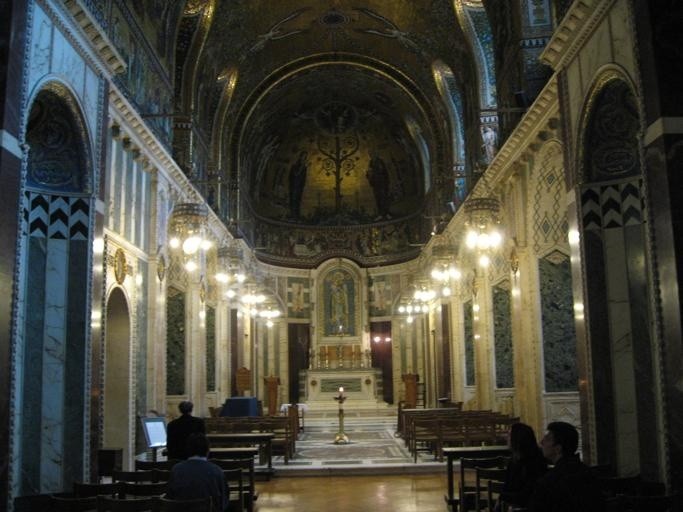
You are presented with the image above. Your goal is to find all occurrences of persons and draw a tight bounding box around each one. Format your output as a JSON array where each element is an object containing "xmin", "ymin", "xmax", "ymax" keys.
[
  {"xmin": 164, "ymin": 431, "xmax": 230, "ymax": 511},
  {"xmin": 540, "ymin": 421, "xmax": 594, "ymax": 512},
  {"xmin": 166, "ymin": 400, "xmax": 205, "ymax": 461},
  {"xmin": 493, "ymin": 422, "xmax": 541, "ymax": 511}
]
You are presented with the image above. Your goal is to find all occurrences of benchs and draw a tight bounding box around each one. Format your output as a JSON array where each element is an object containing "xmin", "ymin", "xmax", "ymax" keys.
[
  {"xmin": 403, "ymin": 410, "xmax": 666, "ymax": 511},
  {"xmin": 13, "ymin": 415, "xmax": 299, "ymax": 512}
]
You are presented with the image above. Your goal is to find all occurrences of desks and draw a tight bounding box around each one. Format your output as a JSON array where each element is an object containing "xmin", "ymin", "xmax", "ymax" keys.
[{"xmin": 283, "ymin": 403, "xmax": 305, "ymax": 433}]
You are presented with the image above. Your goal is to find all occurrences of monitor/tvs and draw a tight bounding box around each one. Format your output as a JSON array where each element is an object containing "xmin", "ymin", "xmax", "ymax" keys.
[{"xmin": 142, "ymin": 417, "xmax": 168, "ymax": 449}]
